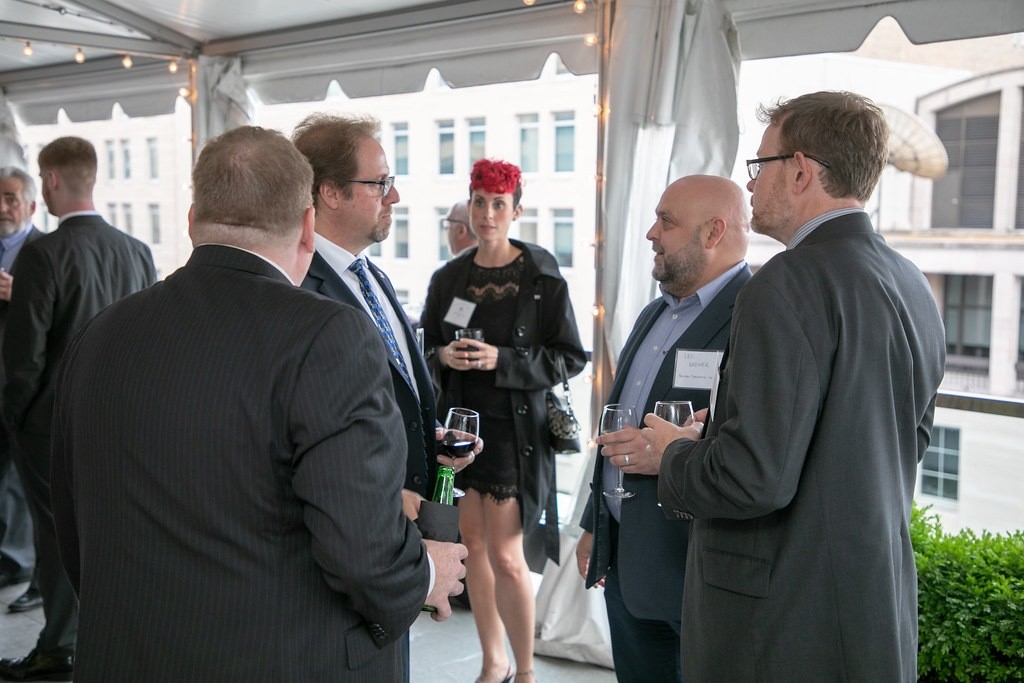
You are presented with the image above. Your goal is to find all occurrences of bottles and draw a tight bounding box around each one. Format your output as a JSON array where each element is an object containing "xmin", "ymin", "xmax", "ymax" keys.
[{"xmin": 417, "ymin": 466, "xmax": 456, "ymax": 611}]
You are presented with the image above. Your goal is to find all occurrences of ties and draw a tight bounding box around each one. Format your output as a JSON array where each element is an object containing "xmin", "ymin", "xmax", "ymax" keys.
[{"xmin": 347, "ymin": 259, "xmax": 413, "ymax": 391}]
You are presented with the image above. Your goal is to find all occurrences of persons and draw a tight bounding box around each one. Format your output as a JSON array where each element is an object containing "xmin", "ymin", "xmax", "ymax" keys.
[
  {"xmin": 640, "ymin": 91, "xmax": 947, "ymax": 683},
  {"xmin": 0, "ymin": 137, "xmax": 158, "ymax": 683},
  {"xmin": 47, "ymin": 124, "xmax": 468, "ymax": 683},
  {"xmin": 575, "ymin": 174, "xmax": 752, "ymax": 683},
  {"xmin": 418, "ymin": 161, "xmax": 586, "ymax": 683},
  {"xmin": 272, "ymin": 116, "xmax": 484, "ymax": 522}
]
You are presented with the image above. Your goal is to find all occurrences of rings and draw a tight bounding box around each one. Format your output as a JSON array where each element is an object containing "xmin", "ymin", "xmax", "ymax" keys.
[
  {"xmin": 645, "ymin": 445, "xmax": 651, "ymax": 453},
  {"xmin": 476, "ymin": 360, "xmax": 481, "ymax": 369},
  {"xmin": 625, "ymin": 455, "xmax": 630, "ymax": 466}
]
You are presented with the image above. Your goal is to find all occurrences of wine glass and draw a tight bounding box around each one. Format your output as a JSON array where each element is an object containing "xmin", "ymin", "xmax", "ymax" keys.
[
  {"xmin": 441, "ymin": 407, "xmax": 479, "ymax": 497},
  {"xmin": 600, "ymin": 404, "xmax": 638, "ymax": 499}
]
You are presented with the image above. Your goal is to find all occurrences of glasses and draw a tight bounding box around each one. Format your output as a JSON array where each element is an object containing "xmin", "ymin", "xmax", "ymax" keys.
[
  {"xmin": 440, "ymin": 218, "xmax": 471, "ymax": 235},
  {"xmin": 347, "ymin": 177, "xmax": 396, "ymax": 199},
  {"xmin": 746, "ymin": 154, "xmax": 830, "ymax": 180}
]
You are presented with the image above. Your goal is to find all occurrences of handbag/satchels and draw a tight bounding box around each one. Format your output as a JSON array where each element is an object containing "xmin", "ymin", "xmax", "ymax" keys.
[{"xmin": 544, "ymin": 350, "xmax": 581, "ymax": 455}]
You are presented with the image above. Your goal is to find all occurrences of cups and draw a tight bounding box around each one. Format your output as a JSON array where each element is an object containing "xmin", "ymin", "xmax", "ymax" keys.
[
  {"xmin": 454, "ymin": 327, "xmax": 483, "ymax": 361},
  {"xmin": 653, "ymin": 399, "xmax": 695, "ymax": 428}
]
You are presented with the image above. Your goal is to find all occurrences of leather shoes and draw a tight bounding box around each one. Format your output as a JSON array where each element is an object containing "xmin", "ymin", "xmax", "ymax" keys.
[
  {"xmin": 8, "ymin": 590, "xmax": 43, "ymax": 611},
  {"xmin": 0, "ymin": 649, "xmax": 75, "ymax": 683}
]
